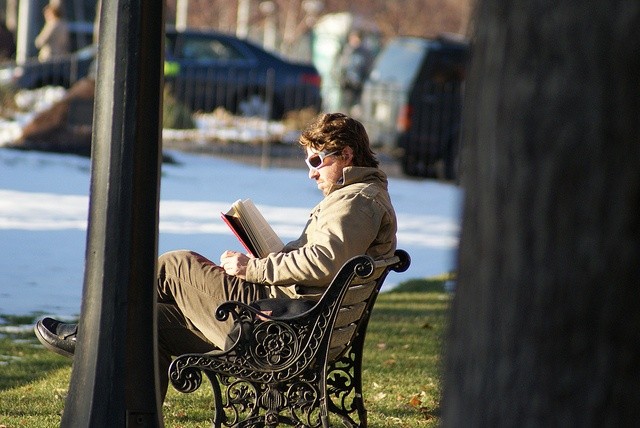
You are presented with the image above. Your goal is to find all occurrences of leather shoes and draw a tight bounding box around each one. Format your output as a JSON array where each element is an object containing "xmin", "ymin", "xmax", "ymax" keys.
[{"xmin": 34, "ymin": 317, "xmax": 78, "ymax": 358}]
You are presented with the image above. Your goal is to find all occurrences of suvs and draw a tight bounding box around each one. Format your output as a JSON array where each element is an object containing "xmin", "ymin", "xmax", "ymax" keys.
[{"xmin": 361, "ymin": 35, "xmax": 471, "ymax": 179}]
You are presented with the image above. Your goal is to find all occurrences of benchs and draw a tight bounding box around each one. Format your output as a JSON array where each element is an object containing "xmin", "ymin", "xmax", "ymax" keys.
[{"xmin": 166, "ymin": 249, "xmax": 412, "ymax": 426}]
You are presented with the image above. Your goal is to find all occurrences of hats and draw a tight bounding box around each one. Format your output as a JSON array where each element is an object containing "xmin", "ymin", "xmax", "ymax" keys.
[{"xmin": 42, "ymin": 0, "xmax": 65, "ymax": 15}]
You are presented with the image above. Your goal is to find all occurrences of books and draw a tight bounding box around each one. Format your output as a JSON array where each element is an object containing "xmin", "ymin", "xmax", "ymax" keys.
[{"xmin": 220, "ymin": 197, "xmax": 287, "ymax": 261}]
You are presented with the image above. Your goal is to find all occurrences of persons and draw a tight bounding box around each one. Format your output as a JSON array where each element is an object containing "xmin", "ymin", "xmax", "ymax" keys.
[
  {"xmin": 33, "ymin": 111, "xmax": 399, "ymax": 420},
  {"xmin": 33, "ymin": 1, "xmax": 71, "ymax": 66},
  {"xmin": 331, "ymin": 24, "xmax": 376, "ymax": 113}
]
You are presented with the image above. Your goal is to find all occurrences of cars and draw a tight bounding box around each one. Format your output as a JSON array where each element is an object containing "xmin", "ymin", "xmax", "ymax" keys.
[{"xmin": 0, "ymin": 30, "xmax": 320, "ymax": 120}]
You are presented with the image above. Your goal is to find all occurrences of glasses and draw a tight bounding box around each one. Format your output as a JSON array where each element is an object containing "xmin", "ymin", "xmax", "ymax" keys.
[{"xmin": 305, "ymin": 148, "xmax": 338, "ymax": 170}]
